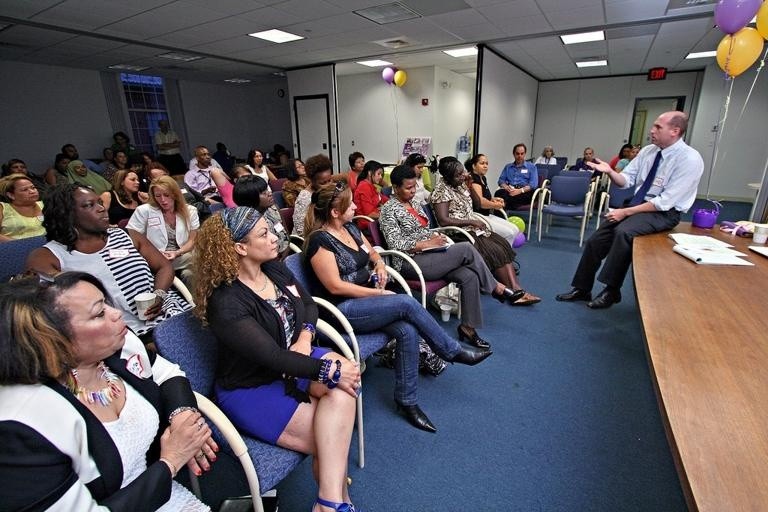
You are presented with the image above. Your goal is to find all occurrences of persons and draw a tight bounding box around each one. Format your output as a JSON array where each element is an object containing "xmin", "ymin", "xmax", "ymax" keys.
[
  {"xmin": 554, "ymin": 110, "xmax": 705, "ymax": 308},
  {"xmin": 2, "ymin": 121, "xmax": 541, "ymax": 511},
  {"xmin": 609, "ymin": 144, "xmax": 633, "ymax": 170},
  {"xmin": 534, "ymin": 146, "xmax": 557, "ymax": 167},
  {"xmin": 574, "ymin": 147, "xmax": 600, "ymax": 219},
  {"xmin": 609, "ymin": 146, "xmax": 640, "ymax": 209}
]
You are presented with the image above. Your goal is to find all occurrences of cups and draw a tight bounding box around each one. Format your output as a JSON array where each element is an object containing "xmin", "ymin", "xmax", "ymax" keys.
[
  {"xmin": 511, "ymin": 186, "xmax": 514, "ymax": 189},
  {"xmin": 439, "ymin": 305, "xmax": 452, "ymax": 322},
  {"xmin": 133, "ymin": 292, "xmax": 157, "ymax": 321}
]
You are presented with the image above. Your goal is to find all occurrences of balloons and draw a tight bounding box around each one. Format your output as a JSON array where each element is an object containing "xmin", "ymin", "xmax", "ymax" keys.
[
  {"xmin": 382, "ymin": 66, "xmax": 407, "ymax": 88},
  {"xmin": 716, "ymin": 27, "xmax": 764, "ymax": 78},
  {"xmin": 713, "ymin": 0, "xmax": 763, "ymax": 35},
  {"xmin": 755, "ymin": 0, "xmax": 767, "ymax": 40}
]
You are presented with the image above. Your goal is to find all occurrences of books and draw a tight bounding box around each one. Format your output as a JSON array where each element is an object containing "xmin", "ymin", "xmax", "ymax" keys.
[{"xmin": 672, "ymin": 245, "xmax": 756, "ymax": 266}]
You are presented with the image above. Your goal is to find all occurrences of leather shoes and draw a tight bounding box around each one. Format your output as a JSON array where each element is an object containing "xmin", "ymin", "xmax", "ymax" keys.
[{"xmin": 556, "ymin": 289, "xmax": 621, "ymax": 309}]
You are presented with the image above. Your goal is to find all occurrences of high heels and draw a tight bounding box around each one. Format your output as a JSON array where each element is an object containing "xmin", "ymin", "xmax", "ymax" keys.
[
  {"xmin": 394, "ymin": 399, "xmax": 436, "ymax": 432},
  {"xmin": 493, "ymin": 286, "xmax": 541, "ymax": 305},
  {"xmin": 451, "ymin": 324, "xmax": 493, "ymax": 366}
]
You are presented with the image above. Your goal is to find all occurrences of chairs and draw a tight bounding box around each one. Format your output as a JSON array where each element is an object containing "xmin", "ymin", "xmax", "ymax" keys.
[{"xmin": 0, "ymin": 154, "xmax": 642, "ymax": 512}]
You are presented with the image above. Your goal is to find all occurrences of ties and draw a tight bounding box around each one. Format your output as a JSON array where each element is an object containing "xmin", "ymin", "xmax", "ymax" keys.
[{"xmin": 629, "ymin": 151, "xmax": 661, "ymax": 206}]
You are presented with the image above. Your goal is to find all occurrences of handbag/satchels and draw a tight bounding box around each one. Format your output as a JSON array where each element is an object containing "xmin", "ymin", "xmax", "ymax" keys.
[{"xmin": 374, "ymin": 333, "xmax": 447, "ymax": 377}]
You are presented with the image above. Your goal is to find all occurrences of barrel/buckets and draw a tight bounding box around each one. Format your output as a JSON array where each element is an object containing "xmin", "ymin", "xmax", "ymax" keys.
[{"xmin": 692, "ymin": 199, "xmax": 719, "ymax": 228}]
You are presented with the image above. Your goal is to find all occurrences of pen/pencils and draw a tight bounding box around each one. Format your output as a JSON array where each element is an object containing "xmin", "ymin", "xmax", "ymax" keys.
[{"xmin": 438, "ymin": 232, "xmax": 449, "ymax": 244}]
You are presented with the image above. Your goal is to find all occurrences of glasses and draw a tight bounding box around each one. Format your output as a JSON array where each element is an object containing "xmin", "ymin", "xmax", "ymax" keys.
[
  {"xmin": 330, "ymin": 179, "xmax": 347, "ymax": 202},
  {"xmin": 23, "ymin": 268, "xmax": 55, "ymax": 289}
]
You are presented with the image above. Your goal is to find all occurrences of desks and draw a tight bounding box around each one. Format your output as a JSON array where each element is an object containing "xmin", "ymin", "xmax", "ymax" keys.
[{"xmin": 627, "ymin": 219, "xmax": 768, "ymax": 512}]
